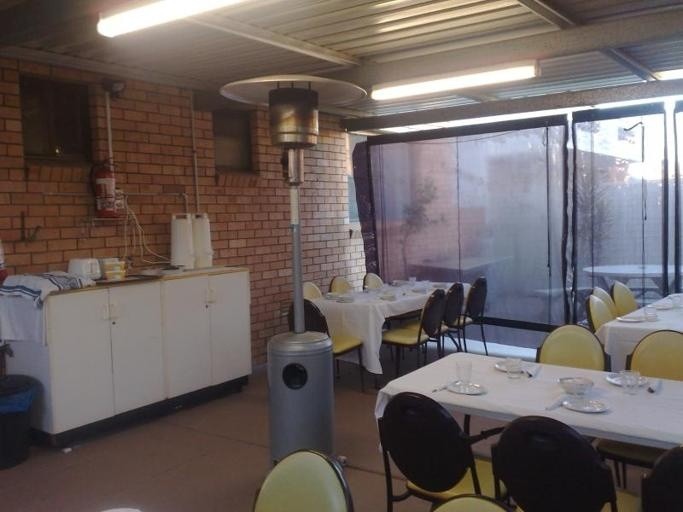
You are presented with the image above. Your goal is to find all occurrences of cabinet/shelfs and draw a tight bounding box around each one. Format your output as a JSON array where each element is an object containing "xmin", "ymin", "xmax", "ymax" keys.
[
  {"xmin": 2, "ymin": 276, "xmax": 162, "ymax": 450},
  {"xmin": 163, "ymin": 270, "xmax": 253, "ymax": 416}
]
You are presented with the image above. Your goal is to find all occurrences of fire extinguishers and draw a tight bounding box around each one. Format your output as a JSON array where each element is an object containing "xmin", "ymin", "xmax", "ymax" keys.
[{"xmin": 89, "ymin": 156, "xmax": 119, "ymax": 218}]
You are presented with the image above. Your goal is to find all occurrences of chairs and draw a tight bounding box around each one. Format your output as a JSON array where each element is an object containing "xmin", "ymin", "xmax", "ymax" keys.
[
  {"xmin": 290, "ymin": 271, "xmax": 486, "ymax": 396},
  {"xmin": 254, "ymin": 450, "xmax": 355, "ymax": 511},
  {"xmin": 630, "ymin": 330, "xmax": 683, "ymax": 381},
  {"xmin": 536, "ymin": 280, "xmax": 637, "ymax": 370}
]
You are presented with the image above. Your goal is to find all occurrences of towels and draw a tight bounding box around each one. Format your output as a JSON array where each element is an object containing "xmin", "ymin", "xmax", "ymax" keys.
[{"xmin": 1, "ymin": 270, "xmax": 96, "ymax": 307}]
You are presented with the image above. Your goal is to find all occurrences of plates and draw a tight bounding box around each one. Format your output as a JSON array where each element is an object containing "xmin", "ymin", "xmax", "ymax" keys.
[
  {"xmin": 563, "ymin": 399, "xmax": 610, "ymax": 413},
  {"xmin": 327, "ymin": 280, "xmax": 447, "ymax": 303},
  {"xmin": 607, "ymin": 373, "xmax": 648, "ymax": 386},
  {"xmin": 616, "ymin": 316, "xmax": 644, "ymax": 322},
  {"xmin": 494, "ymin": 360, "xmax": 522, "ymax": 374},
  {"xmin": 448, "ymin": 383, "xmax": 487, "ymax": 395}
]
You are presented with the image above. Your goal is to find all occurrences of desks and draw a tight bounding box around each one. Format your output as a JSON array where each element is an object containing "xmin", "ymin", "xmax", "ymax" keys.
[
  {"xmin": 584, "ymin": 264, "xmax": 682, "ymax": 305},
  {"xmin": 411, "ymin": 254, "xmax": 512, "ymax": 283}
]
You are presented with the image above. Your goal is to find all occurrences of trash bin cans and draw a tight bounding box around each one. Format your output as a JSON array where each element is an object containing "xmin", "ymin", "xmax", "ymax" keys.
[{"xmin": 0, "ymin": 374, "xmax": 38, "ymax": 469}]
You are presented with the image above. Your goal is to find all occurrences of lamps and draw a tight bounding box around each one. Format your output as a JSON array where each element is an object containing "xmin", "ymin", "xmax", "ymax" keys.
[
  {"xmin": 97, "ymin": 0, "xmax": 246, "ymax": 40},
  {"xmin": 369, "ymin": 57, "xmax": 541, "ymax": 104}
]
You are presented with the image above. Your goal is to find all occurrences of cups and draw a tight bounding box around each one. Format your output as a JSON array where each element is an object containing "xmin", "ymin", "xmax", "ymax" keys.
[
  {"xmin": 507, "ymin": 357, "xmax": 522, "ymax": 379},
  {"xmin": 456, "ymin": 361, "xmax": 472, "ymax": 383},
  {"xmin": 558, "ymin": 377, "xmax": 594, "ymax": 408},
  {"xmin": 645, "ymin": 305, "xmax": 656, "ymax": 320},
  {"xmin": 620, "ymin": 368, "xmax": 639, "ymax": 394}
]
